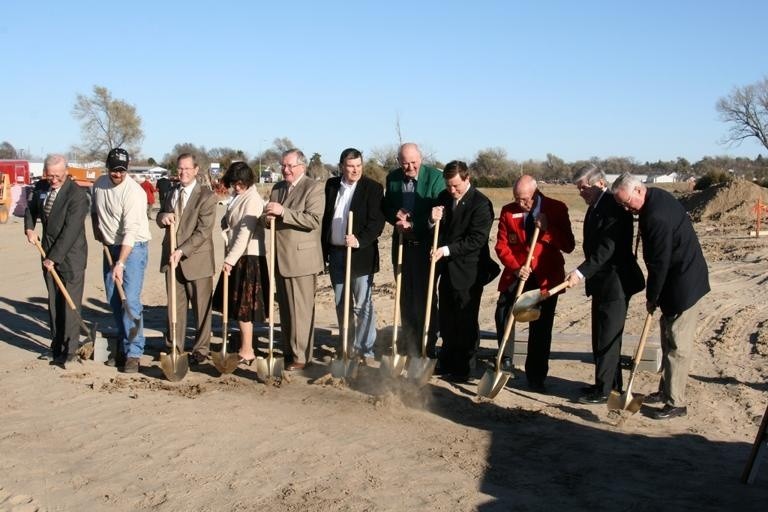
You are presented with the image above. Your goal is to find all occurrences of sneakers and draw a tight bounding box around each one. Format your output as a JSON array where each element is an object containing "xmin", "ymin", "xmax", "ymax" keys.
[
  {"xmin": 122, "ymin": 356, "xmax": 141, "ymax": 374},
  {"xmin": 103, "ymin": 353, "xmax": 124, "ymax": 368}
]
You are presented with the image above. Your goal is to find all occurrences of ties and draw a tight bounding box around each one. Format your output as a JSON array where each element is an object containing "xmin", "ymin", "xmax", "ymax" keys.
[
  {"xmin": 43, "ymin": 188, "xmax": 57, "ymax": 224},
  {"xmin": 284, "ymin": 183, "xmax": 295, "ymax": 199},
  {"xmin": 524, "ymin": 209, "xmax": 537, "ymax": 248},
  {"xmin": 454, "ymin": 199, "xmax": 460, "ymax": 210},
  {"xmin": 404, "ymin": 178, "xmax": 415, "ymax": 210},
  {"xmin": 634, "ymin": 222, "xmax": 642, "ymax": 261},
  {"xmin": 173, "ymin": 189, "xmax": 186, "ymax": 249}
]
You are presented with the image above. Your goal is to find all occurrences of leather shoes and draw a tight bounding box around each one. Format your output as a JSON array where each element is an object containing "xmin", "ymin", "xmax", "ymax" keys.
[
  {"xmin": 576, "ymin": 384, "xmax": 623, "ymax": 405},
  {"xmin": 37, "ymin": 350, "xmax": 80, "ymax": 369},
  {"xmin": 528, "ymin": 380, "xmax": 547, "ymax": 394},
  {"xmin": 643, "ymin": 390, "xmax": 665, "ymax": 404},
  {"xmin": 651, "ymin": 403, "xmax": 687, "ymax": 421},
  {"xmin": 189, "ymin": 351, "xmax": 211, "ymax": 370},
  {"xmin": 500, "ymin": 357, "xmax": 520, "ymax": 382},
  {"xmin": 286, "ymin": 362, "xmax": 314, "ymax": 371},
  {"xmin": 237, "ymin": 353, "xmax": 255, "ymax": 366}
]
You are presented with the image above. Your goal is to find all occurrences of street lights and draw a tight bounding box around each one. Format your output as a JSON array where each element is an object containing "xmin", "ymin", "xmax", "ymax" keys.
[{"xmin": 258, "ymin": 138, "xmax": 267, "ymax": 182}]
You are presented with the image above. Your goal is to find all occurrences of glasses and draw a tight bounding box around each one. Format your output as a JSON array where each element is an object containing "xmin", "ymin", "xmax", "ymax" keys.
[
  {"xmin": 45, "ymin": 172, "xmax": 65, "ymax": 180},
  {"xmin": 176, "ymin": 167, "xmax": 194, "ymax": 173},
  {"xmin": 281, "ymin": 162, "xmax": 302, "ymax": 170},
  {"xmin": 617, "ymin": 189, "xmax": 635, "ymax": 208},
  {"xmin": 230, "ymin": 182, "xmax": 249, "ymax": 191},
  {"xmin": 512, "ymin": 192, "xmax": 536, "ymax": 205}
]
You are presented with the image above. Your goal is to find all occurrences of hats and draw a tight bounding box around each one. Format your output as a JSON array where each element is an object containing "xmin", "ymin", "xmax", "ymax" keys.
[{"xmin": 105, "ymin": 148, "xmax": 129, "ymax": 171}]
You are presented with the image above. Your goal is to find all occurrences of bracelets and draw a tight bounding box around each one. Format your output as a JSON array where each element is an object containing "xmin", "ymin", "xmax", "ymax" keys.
[
  {"xmin": 176, "ymin": 249, "xmax": 184, "ymax": 255},
  {"xmin": 116, "ymin": 262, "xmax": 126, "ymax": 270}
]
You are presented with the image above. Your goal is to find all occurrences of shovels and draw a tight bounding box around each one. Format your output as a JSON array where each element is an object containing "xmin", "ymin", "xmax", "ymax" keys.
[
  {"xmin": 477, "ymin": 220, "xmax": 571, "ymax": 399},
  {"xmin": 36, "ymin": 241, "xmax": 97, "ymax": 358},
  {"xmin": 381, "ymin": 229, "xmax": 407, "ymax": 380},
  {"xmin": 406, "ymin": 218, "xmax": 440, "ymax": 389},
  {"xmin": 329, "ymin": 212, "xmax": 359, "ymax": 381},
  {"xmin": 212, "ymin": 271, "xmax": 239, "ymax": 374},
  {"xmin": 160, "ymin": 221, "xmax": 189, "ymax": 381},
  {"xmin": 607, "ymin": 314, "xmax": 652, "ymax": 415},
  {"xmin": 101, "ymin": 244, "xmax": 142, "ymax": 346},
  {"xmin": 255, "ymin": 216, "xmax": 285, "ymax": 382},
  {"xmin": 513, "ymin": 275, "xmax": 569, "ymax": 322}
]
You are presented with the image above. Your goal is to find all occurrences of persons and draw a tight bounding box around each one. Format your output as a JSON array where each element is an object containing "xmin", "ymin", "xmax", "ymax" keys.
[
  {"xmin": 213, "ymin": 160, "xmax": 270, "ymax": 365},
  {"xmin": 261, "ymin": 149, "xmax": 325, "ymax": 370},
  {"xmin": 565, "ymin": 164, "xmax": 646, "ymax": 403},
  {"xmin": 428, "ymin": 160, "xmax": 500, "ymax": 382},
  {"xmin": 156, "ymin": 172, "xmax": 171, "ymax": 209},
  {"xmin": 479, "ymin": 174, "xmax": 575, "ymax": 393},
  {"xmin": 321, "ymin": 148, "xmax": 386, "ymax": 366},
  {"xmin": 92, "ymin": 148, "xmax": 150, "ymax": 374},
  {"xmin": 24, "ymin": 153, "xmax": 90, "ymax": 369},
  {"xmin": 140, "ymin": 176, "xmax": 159, "ymax": 220},
  {"xmin": 157, "ymin": 153, "xmax": 219, "ymax": 364},
  {"xmin": 385, "ymin": 145, "xmax": 447, "ymax": 358},
  {"xmin": 611, "ymin": 171, "xmax": 710, "ymax": 420}
]
